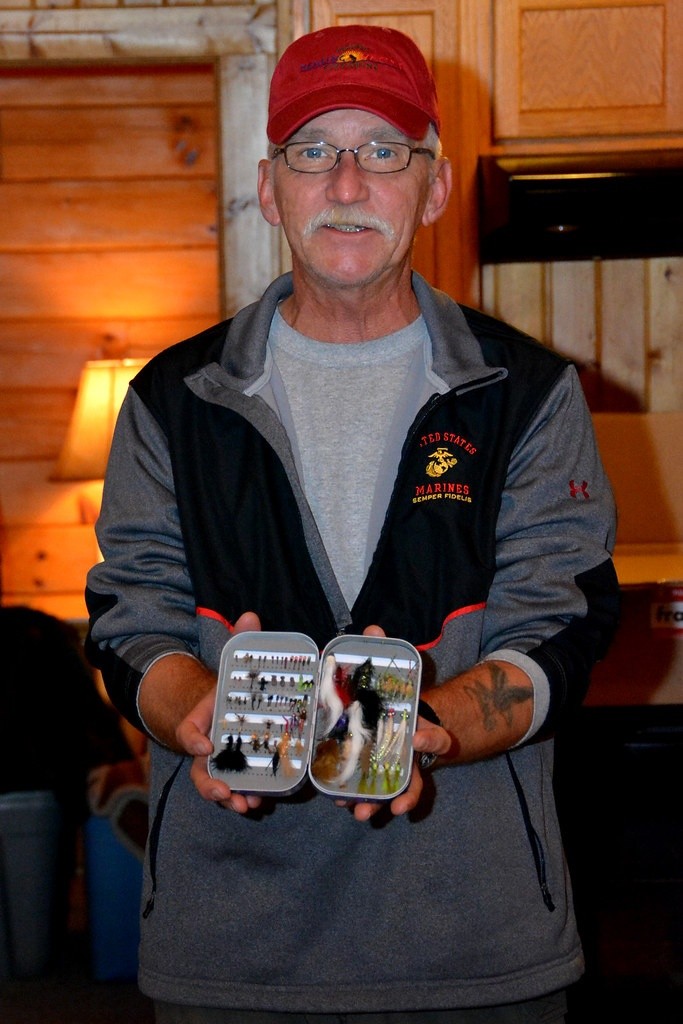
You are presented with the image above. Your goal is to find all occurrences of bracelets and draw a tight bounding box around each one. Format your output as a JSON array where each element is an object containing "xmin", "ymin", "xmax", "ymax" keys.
[{"xmin": 418, "ymin": 698, "xmax": 442, "ymax": 770}]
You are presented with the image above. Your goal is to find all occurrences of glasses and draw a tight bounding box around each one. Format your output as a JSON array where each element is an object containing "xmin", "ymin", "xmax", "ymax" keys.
[{"xmin": 272, "ymin": 141, "xmax": 435, "ymax": 173}]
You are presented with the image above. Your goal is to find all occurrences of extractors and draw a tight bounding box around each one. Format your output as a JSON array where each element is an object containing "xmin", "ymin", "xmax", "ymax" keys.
[{"xmin": 478, "ymin": 145, "xmax": 683, "ymax": 266}]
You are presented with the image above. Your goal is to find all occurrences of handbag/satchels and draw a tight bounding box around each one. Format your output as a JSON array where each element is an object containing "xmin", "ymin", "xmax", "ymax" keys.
[{"xmin": 0, "ymin": 606, "xmax": 134, "ymax": 835}]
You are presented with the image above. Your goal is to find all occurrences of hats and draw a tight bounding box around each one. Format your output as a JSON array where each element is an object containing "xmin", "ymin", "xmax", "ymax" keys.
[{"xmin": 267, "ymin": 25, "xmax": 441, "ymax": 145}]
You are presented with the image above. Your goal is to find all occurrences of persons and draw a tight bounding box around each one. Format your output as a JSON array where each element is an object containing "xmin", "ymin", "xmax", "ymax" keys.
[{"xmin": 84, "ymin": 25, "xmax": 624, "ymax": 1023}]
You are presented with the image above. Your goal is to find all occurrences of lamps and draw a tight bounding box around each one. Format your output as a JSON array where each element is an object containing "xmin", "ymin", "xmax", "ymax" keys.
[{"xmin": 49, "ymin": 359, "xmax": 156, "ymax": 562}]
